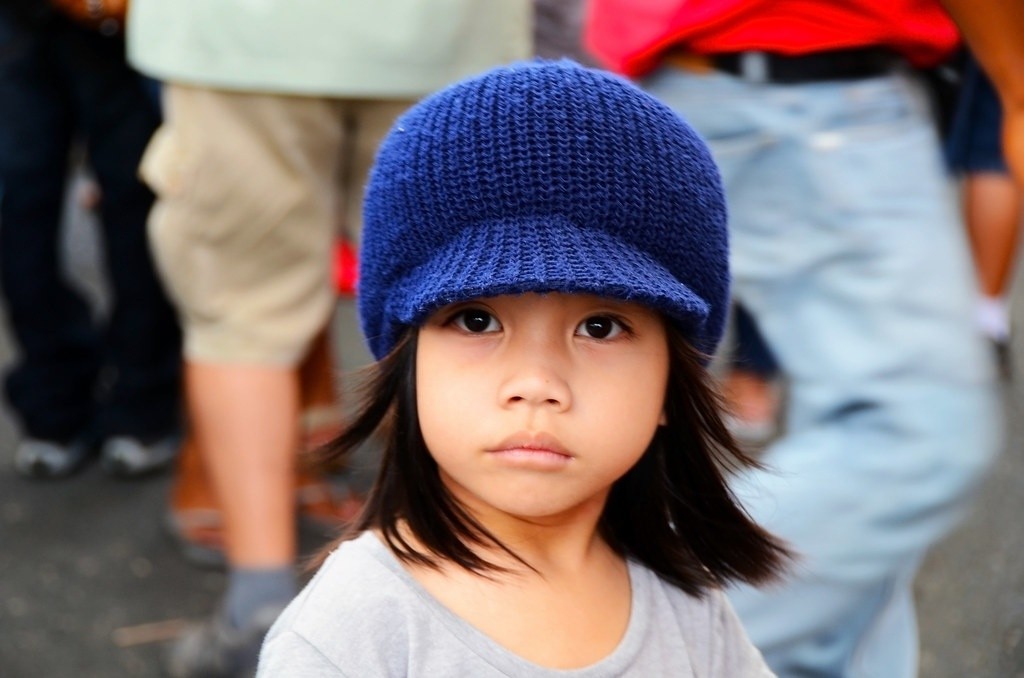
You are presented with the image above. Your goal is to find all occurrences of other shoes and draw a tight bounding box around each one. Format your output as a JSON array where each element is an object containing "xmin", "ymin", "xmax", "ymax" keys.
[
  {"xmin": 107, "ymin": 420, "xmax": 182, "ymax": 472},
  {"xmin": 18, "ymin": 426, "xmax": 97, "ymax": 479},
  {"xmin": 156, "ymin": 580, "xmax": 305, "ymax": 677}
]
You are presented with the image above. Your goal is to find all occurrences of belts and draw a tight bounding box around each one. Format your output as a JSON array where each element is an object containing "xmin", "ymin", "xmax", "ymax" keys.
[{"xmin": 699, "ymin": 39, "xmax": 894, "ymax": 87}]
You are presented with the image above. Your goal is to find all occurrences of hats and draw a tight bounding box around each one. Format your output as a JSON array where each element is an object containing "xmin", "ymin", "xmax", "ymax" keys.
[{"xmin": 355, "ymin": 62, "xmax": 732, "ymax": 381}]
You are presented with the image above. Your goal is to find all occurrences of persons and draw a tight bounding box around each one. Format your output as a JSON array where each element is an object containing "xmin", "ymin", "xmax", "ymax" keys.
[
  {"xmin": 0, "ymin": 1, "xmax": 578, "ymax": 678},
  {"xmin": 583, "ymin": 2, "xmax": 1022, "ymax": 678},
  {"xmin": 256, "ymin": 58, "xmax": 793, "ymax": 677}
]
